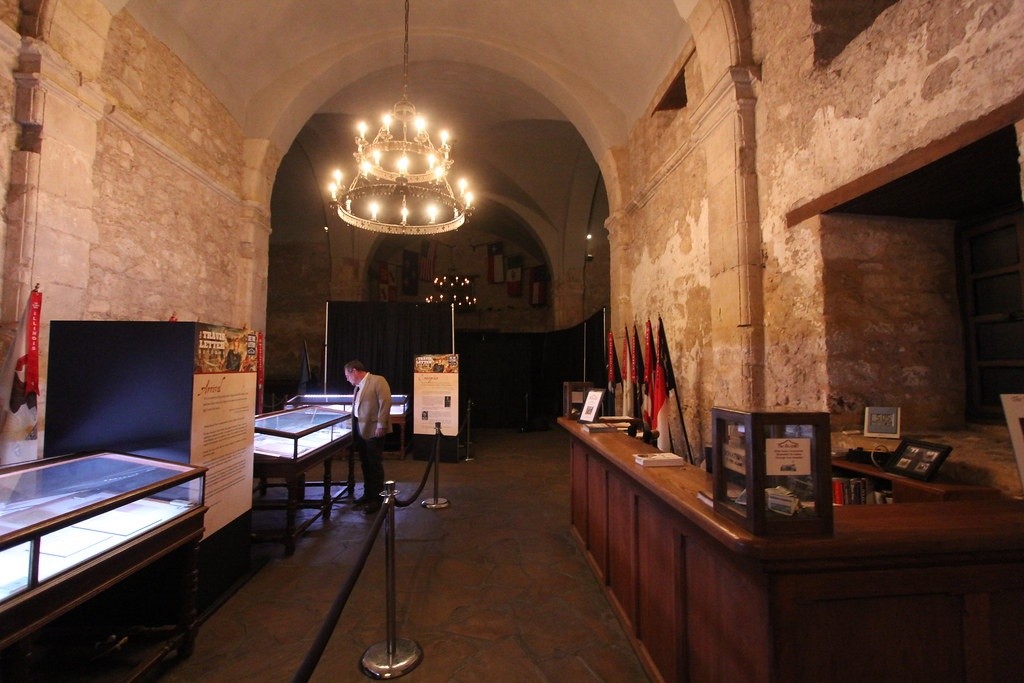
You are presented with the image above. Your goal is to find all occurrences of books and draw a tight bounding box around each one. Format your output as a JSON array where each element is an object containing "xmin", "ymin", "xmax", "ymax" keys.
[
  {"xmin": 832, "ymin": 475, "xmax": 874, "ymax": 506},
  {"xmin": 632, "ymin": 452, "xmax": 684, "ymax": 466},
  {"xmin": 698, "ymin": 488, "xmax": 745, "ymax": 508},
  {"xmin": 583, "ymin": 423, "xmax": 617, "ymax": 432}
]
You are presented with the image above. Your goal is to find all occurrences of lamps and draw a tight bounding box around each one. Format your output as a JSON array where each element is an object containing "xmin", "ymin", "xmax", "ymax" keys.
[
  {"xmin": 423, "ymin": 237, "xmax": 479, "ymax": 307},
  {"xmin": 328, "ymin": 0, "xmax": 475, "ymax": 236}
]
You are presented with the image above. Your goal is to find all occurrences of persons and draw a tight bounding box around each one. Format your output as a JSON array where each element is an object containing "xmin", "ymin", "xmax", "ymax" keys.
[
  {"xmin": 225, "ymin": 341, "xmax": 242, "ymax": 371},
  {"xmin": 344, "ymin": 360, "xmax": 394, "ymax": 516}
]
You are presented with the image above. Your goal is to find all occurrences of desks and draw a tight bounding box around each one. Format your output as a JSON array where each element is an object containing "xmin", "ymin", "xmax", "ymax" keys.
[{"xmin": 557, "ymin": 415, "xmax": 1024, "ymax": 683}]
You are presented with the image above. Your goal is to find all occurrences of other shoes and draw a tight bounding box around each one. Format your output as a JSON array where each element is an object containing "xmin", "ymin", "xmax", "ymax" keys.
[
  {"xmin": 352, "ymin": 495, "xmax": 371, "ymax": 504},
  {"xmin": 364, "ymin": 501, "xmax": 382, "ymax": 511}
]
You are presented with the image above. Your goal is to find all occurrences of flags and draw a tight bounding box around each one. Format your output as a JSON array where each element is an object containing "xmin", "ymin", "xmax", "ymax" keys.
[
  {"xmin": 378, "ymin": 261, "xmax": 399, "ymax": 302},
  {"xmin": 506, "ymin": 256, "xmax": 524, "ymax": 298},
  {"xmin": 529, "ymin": 265, "xmax": 548, "ymax": 308},
  {"xmin": 487, "ymin": 241, "xmax": 504, "ymax": 284},
  {"xmin": 607, "ymin": 317, "xmax": 688, "ymax": 466},
  {"xmin": 0, "ymin": 291, "xmax": 42, "ymax": 508},
  {"xmin": 402, "ymin": 250, "xmax": 419, "ymax": 295},
  {"xmin": 298, "ymin": 345, "xmax": 311, "ymax": 394},
  {"xmin": 417, "ymin": 238, "xmax": 436, "ymax": 280}
]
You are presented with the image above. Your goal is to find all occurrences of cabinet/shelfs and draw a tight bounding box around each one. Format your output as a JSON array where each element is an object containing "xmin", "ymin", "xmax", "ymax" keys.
[{"xmin": 830, "ymin": 460, "xmax": 1001, "ymax": 505}]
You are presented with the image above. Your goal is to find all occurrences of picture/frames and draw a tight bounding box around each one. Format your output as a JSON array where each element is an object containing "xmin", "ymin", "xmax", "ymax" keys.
[{"xmin": 883, "ymin": 438, "xmax": 952, "ymax": 481}]
